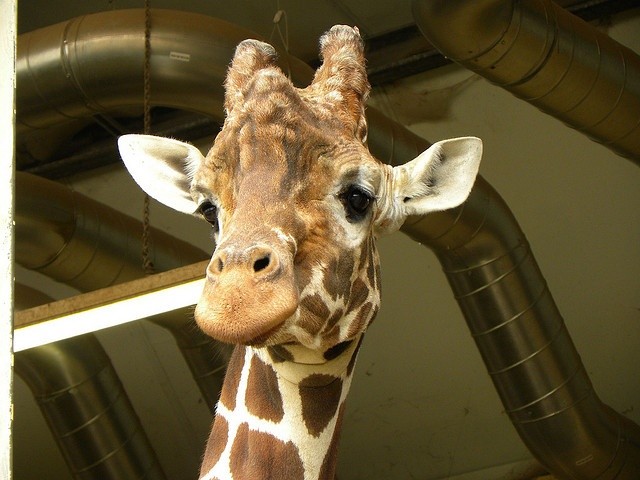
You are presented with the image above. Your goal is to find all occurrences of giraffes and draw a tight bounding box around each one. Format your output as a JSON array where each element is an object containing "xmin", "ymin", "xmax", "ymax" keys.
[{"xmin": 117, "ymin": 24, "xmax": 484, "ymax": 480}]
